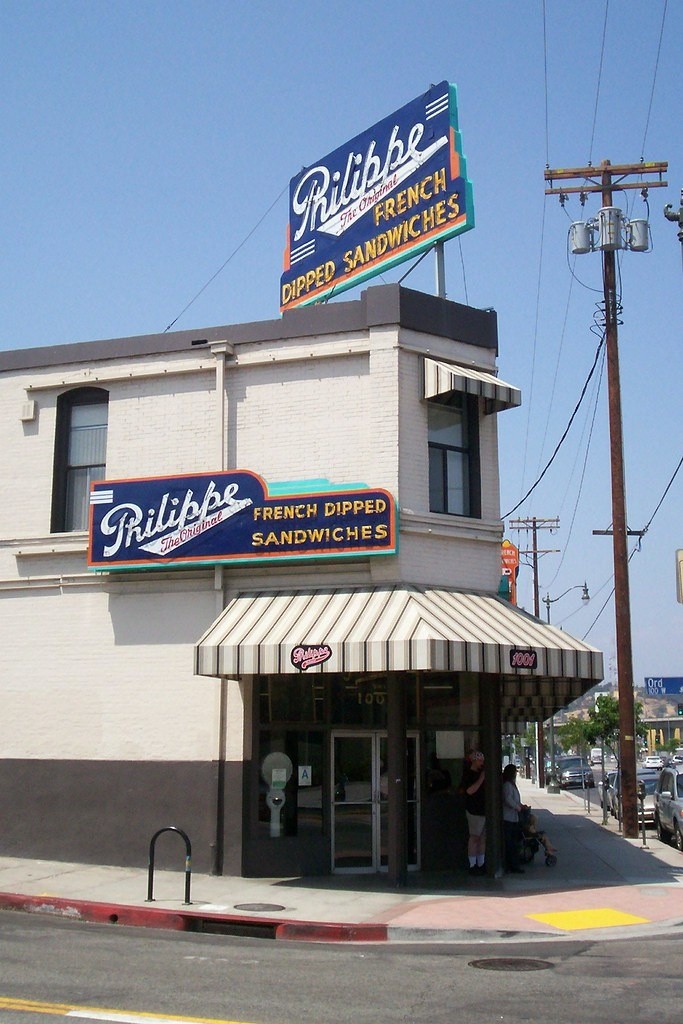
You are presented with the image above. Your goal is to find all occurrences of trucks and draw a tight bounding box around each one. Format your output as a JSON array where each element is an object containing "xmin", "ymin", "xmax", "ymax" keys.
[{"xmin": 591, "ymin": 748, "xmax": 606, "ymax": 765}]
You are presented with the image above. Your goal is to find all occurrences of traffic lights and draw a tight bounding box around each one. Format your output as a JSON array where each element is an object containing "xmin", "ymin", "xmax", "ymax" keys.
[{"xmin": 677, "ymin": 703, "xmax": 683, "ymax": 715}]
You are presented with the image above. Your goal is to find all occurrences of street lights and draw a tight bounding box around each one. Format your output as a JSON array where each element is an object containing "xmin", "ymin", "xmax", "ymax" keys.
[{"xmin": 539, "ymin": 580, "xmax": 592, "ymax": 795}]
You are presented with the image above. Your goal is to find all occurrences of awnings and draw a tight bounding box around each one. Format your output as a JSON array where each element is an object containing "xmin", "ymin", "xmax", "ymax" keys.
[{"xmin": 197, "ymin": 592, "xmax": 603, "ymax": 734}]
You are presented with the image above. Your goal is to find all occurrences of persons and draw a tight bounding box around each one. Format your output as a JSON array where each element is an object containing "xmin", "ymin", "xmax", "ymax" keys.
[
  {"xmin": 461, "ymin": 751, "xmax": 487, "ymax": 876},
  {"xmin": 502, "ymin": 764, "xmax": 525, "ymax": 873},
  {"xmin": 528, "ymin": 814, "xmax": 557, "ymax": 855}
]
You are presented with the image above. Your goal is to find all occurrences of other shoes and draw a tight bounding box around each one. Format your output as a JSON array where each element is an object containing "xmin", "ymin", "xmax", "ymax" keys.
[
  {"xmin": 470, "ymin": 864, "xmax": 482, "ymax": 877},
  {"xmin": 546, "ymin": 847, "xmax": 556, "ymax": 851},
  {"xmin": 480, "ymin": 863, "xmax": 493, "ymax": 876}
]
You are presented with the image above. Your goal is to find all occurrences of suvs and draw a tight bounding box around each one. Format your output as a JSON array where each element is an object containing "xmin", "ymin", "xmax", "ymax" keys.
[{"xmin": 654, "ymin": 759, "xmax": 683, "ymax": 853}]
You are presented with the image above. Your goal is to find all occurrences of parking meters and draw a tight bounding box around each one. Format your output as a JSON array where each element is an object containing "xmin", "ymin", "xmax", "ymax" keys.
[
  {"xmin": 584, "ymin": 771, "xmax": 593, "ymax": 813},
  {"xmin": 636, "ymin": 780, "xmax": 647, "ymax": 846},
  {"xmin": 601, "ymin": 771, "xmax": 610, "ymax": 823}
]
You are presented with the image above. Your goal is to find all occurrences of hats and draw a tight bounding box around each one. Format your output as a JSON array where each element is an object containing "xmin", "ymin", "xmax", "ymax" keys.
[{"xmin": 467, "ymin": 752, "xmax": 484, "ymax": 762}]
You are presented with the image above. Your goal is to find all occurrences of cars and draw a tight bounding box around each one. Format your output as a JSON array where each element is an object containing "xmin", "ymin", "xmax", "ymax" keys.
[
  {"xmin": 544, "ymin": 755, "xmax": 594, "ymax": 790},
  {"xmin": 599, "ymin": 769, "xmax": 661, "ymax": 825},
  {"xmin": 661, "ymin": 755, "xmax": 683, "ymax": 767},
  {"xmin": 640, "ymin": 756, "xmax": 664, "ymax": 770}
]
